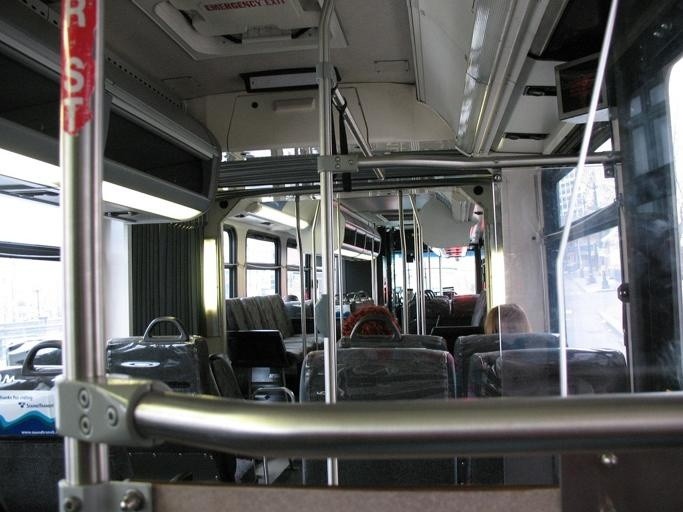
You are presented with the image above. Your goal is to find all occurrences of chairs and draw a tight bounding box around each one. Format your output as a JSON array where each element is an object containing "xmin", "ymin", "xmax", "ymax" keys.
[{"xmin": 0, "ymin": 297, "xmax": 631, "ymax": 512}]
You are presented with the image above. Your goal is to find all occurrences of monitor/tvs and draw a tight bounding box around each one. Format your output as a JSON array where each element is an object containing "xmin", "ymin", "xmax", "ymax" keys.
[{"xmin": 554, "ymin": 52, "xmax": 609, "ymax": 121}]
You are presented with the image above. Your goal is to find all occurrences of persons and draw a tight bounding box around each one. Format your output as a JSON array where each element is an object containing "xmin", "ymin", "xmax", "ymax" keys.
[{"xmin": 483, "ymin": 303, "xmax": 529, "ymax": 334}]
